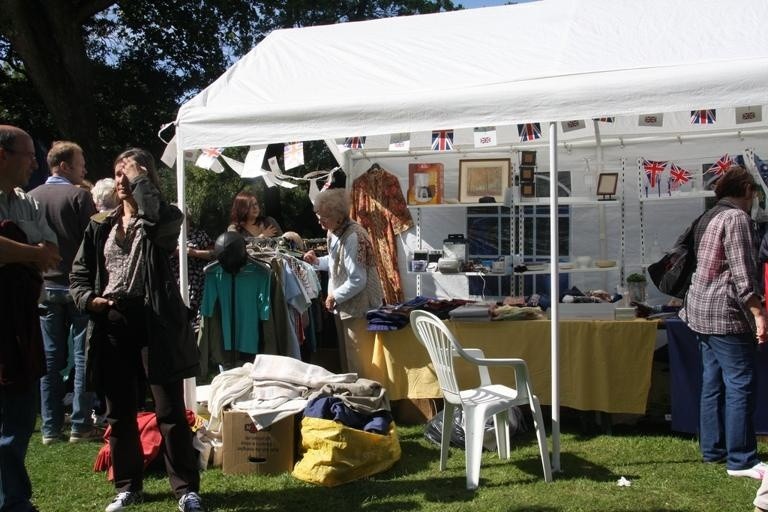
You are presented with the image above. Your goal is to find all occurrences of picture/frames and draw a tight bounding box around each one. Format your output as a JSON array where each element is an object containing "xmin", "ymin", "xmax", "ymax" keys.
[
  {"xmin": 595, "ymin": 170, "xmax": 618, "ymax": 196},
  {"xmin": 517, "ymin": 146, "xmax": 538, "ymax": 196},
  {"xmin": 456, "ymin": 158, "xmax": 511, "ymax": 202}
]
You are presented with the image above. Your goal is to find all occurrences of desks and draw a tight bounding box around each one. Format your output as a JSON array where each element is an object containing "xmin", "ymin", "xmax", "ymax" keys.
[
  {"xmin": 352, "ymin": 299, "xmax": 659, "ymax": 442},
  {"xmin": 654, "ymin": 311, "xmax": 767, "ymax": 436}
]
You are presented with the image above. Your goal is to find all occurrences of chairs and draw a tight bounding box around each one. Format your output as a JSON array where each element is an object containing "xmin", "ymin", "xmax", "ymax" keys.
[{"xmin": 404, "ymin": 308, "xmax": 555, "ymax": 492}]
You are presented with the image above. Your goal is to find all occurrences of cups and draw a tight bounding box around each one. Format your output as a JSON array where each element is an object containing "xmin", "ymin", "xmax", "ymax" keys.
[
  {"xmin": 577, "ymin": 255, "xmax": 592, "ymax": 269},
  {"xmin": 482, "ymin": 260, "xmax": 504, "ymax": 273}
]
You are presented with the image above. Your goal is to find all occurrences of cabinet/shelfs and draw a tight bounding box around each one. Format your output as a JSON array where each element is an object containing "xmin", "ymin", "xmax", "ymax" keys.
[
  {"xmin": 515, "ymin": 193, "xmax": 624, "ymax": 298},
  {"xmin": 405, "ymin": 188, "xmax": 516, "ymax": 300},
  {"xmin": 636, "ymin": 187, "xmax": 723, "ymax": 302}
]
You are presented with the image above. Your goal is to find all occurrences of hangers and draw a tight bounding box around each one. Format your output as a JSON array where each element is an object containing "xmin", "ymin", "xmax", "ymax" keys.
[
  {"xmin": 366, "ymin": 162, "xmax": 381, "ymax": 174},
  {"xmin": 245, "ymin": 237, "xmax": 328, "ymax": 269}
]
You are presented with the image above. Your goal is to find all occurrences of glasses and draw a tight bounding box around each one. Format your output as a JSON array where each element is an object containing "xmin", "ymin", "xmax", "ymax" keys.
[
  {"xmin": 7, "ymin": 147, "xmax": 37, "ymax": 161},
  {"xmin": 316, "ymin": 211, "xmax": 332, "ymax": 223}
]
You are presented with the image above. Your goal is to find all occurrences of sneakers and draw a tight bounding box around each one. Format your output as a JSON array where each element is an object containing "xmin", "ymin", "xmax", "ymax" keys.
[
  {"xmin": 91, "ymin": 401, "xmax": 110, "ymax": 426},
  {"xmin": 70, "ymin": 426, "xmax": 105, "ymax": 442},
  {"xmin": 42, "ymin": 434, "xmax": 70, "ymax": 445},
  {"xmin": 178, "ymin": 491, "xmax": 206, "ymax": 512},
  {"xmin": 105, "ymin": 489, "xmax": 144, "ymax": 511},
  {"xmin": 726, "ymin": 460, "xmax": 767, "ymax": 481}
]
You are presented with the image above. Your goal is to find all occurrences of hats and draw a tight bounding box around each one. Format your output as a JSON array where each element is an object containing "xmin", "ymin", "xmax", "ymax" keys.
[
  {"xmin": 214, "ymin": 232, "xmax": 246, "ymax": 274},
  {"xmin": 716, "ymin": 166, "xmax": 763, "ymax": 199}
]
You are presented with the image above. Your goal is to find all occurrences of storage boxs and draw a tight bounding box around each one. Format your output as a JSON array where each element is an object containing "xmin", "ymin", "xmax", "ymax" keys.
[
  {"xmin": 406, "ymin": 162, "xmax": 444, "ymax": 206},
  {"xmin": 219, "ymin": 405, "xmax": 297, "ymax": 479}
]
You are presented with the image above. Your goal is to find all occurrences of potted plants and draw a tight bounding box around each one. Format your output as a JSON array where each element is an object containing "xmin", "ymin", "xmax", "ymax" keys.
[{"xmin": 625, "ymin": 270, "xmax": 647, "ymax": 301}]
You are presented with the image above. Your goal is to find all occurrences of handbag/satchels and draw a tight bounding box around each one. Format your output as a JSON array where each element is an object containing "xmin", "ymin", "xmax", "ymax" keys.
[{"xmin": 648, "ymin": 211, "xmax": 711, "ymax": 299}]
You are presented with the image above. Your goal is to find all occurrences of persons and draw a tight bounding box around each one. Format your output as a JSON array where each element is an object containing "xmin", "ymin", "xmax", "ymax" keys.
[
  {"xmin": 2, "ymin": 123, "xmax": 205, "ymax": 512},
  {"xmin": 168, "ymin": 188, "xmax": 385, "ymax": 388},
  {"xmin": 679, "ymin": 166, "xmax": 768, "ymax": 481}
]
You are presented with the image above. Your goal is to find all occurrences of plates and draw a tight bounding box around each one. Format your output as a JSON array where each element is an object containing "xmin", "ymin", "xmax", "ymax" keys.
[
  {"xmin": 596, "ymin": 260, "xmax": 616, "ymax": 267},
  {"xmin": 526, "ymin": 265, "xmax": 545, "ymax": 270},
  {"xmin": 559, "ymin": 264, "xmax": 574, "ymax": 269}
]
